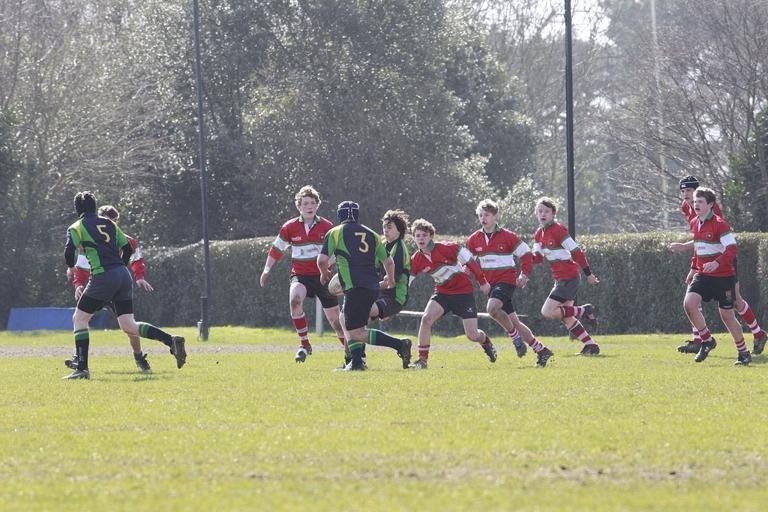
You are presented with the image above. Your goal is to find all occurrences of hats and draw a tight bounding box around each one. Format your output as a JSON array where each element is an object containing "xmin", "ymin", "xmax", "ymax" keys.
[{"xmin": 680, "ymin": 176, "xmax": 700, "ymax": 189}]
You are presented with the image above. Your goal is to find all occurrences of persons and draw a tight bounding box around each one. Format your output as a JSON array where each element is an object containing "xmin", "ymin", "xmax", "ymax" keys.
[
  {"xmin": 684, "ymin": 187, "xmax": 752, "ymax": 365},
  {"xmin": 462, "ymin": 199, "xmax": 554, "ymax": 366},
  {"xmin": 260, "ymin": 187, "xmax": 345, "ymax": 360},
  {"xmin": 370, "ymin": 210, "xmax": 410, "ymax": 319},
  {"xmin": 669, "ymin": 176, "xmax": 768, "ymax": 355},
  {"xmin": 65, "ymin": 205, "xmax": 187, "ymax": 369},
  {"xmin": 317, "ymin": 202, "xmax": 412, "ymax": 371},
  {"xmin": 531, "ymin": 197, "xmax": 600, "ymax": 354},
  {"xmin": 409, "ymin": 219, "xmax": 497, "ymax": 367},
  {"xmin": 63, "ymin": 192, "xmax": 151, "ymax": 378}
]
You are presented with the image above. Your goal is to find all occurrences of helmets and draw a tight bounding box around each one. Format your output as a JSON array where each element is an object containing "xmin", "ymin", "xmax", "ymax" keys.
[
  {"xmin": 73, "ymin": 194, "xmax": 101, "ymax": 213},
  {"xmin": 336, "ymin": 201, "xmax": 361, "ymax": 223}
]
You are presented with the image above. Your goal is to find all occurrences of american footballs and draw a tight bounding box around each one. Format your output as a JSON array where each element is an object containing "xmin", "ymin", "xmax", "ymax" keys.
[{"xmin": 328, "ymin": 272, "xmax": 349, "ymax": 296}]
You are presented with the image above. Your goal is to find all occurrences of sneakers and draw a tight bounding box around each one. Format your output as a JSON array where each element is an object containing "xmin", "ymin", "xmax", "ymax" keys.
[
  {"xmin": 295, "ymin": 345, "xmax": 311, "ymax": 363},
  {"xmin": 399, "ymin": 339, "xmax": 412, "ymax": 367},
  {"xmin": 336, "ymin": 360, "xmax": 368, "ymax": 372},
  {"xmin": 409, "ymin": 359, "xmax": 429, "ymax": 369},
  {"xmin": 735, "ymin": 350, "xmax": 752, "ymax": 365},
  {"xmin": 751, "ymin": 332, "xmax": 768, "ymax": 353},
  {"xmin": 65, "ymin": 356, "xmax": 79, "ymax": 368},
  {"xmin": 576, "ymin": 344, "xmax": 600, "ymax": 357},
  {"xmin": 537, "ymin": 348, "xmax": 554, "ymax": 368},
  {"xmin": 511, "ymin": 338, "xmax": 528, "ymax": 357},
  {"xmin": 696, "ymin": 338, "xmax": 717, "ymax": 362},
  {"xmin": 678, "ymin": 340, "xmax": 702, "ymax": 352},
  {"xmin": 484, "ymin": 337, "xmax": 498, "ymax": 361},
  {"xmin": 584, "ymin": 304, "xmax": 598, "ymax": 330},
  {"xmin": 171, "ymin": 336, "xmax": 187, "ymax": 368}
]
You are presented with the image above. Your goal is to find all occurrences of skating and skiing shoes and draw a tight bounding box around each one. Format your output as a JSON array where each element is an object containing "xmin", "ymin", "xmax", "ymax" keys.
[
  {"xmin": 131, "ymin": 354, "xmax": 153, "ymax": 375},
  {"xmin": 62, "ymin": 369, "xmax": 91, "ymax": 381}
]
